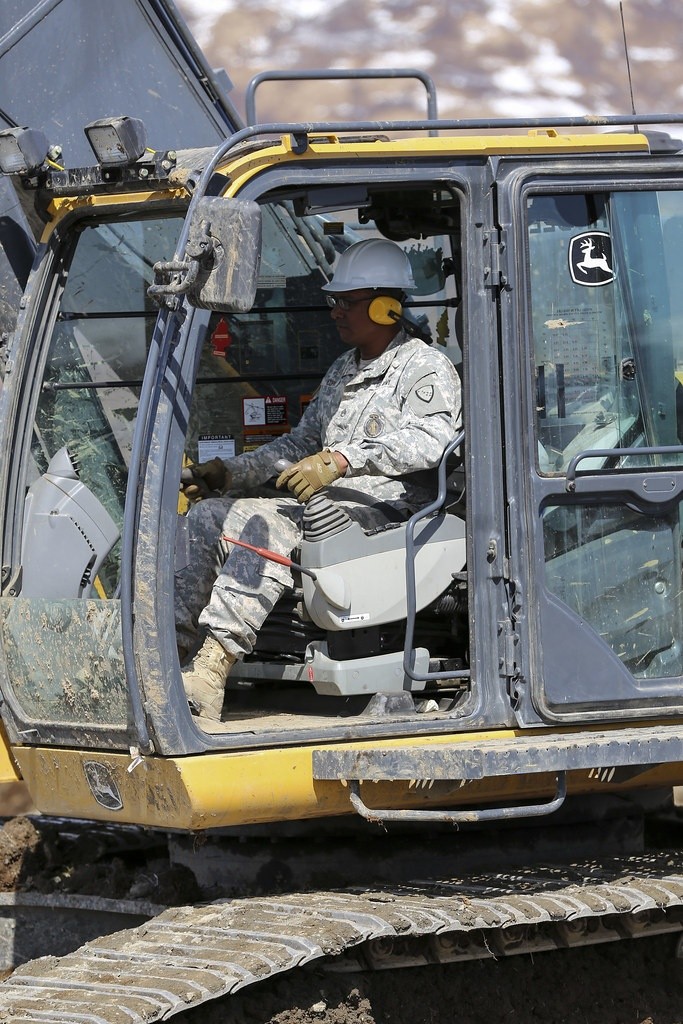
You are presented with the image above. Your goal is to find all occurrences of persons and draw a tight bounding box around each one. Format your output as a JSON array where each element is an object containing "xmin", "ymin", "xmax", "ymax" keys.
[{"xmin": 170, "ymin": 238, "xmax": 462, "ymax": 719}]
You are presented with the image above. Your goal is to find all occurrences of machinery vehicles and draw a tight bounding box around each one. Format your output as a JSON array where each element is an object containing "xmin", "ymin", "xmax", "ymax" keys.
[{"xmin": 1, "ymin": 72, "xmax": 683, "ymax": 1022}]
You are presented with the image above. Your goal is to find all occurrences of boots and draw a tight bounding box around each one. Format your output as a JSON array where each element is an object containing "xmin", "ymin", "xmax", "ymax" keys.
[{"xmin": 180, "ymin": 632, "xmax": 237, "ymax": 723}]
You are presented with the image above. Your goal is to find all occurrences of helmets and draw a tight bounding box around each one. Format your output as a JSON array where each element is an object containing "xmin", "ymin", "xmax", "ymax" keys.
[{"xmin": 321, "ymin": 238, "xmax": 418, "ymax": 292}]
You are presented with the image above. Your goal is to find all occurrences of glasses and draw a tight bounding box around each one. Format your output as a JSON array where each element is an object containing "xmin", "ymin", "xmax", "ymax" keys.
[{"xmin": 325, "ymin": 294, "xmax": 378, "ymax": 311}]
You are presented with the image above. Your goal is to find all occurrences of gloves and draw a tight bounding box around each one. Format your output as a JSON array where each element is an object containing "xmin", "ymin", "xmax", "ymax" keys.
[
  {"xmin": 183, "ymin": 456, "xmax": 225, "ymax": 503},
  {"xmin": 276, "ymin": 448, "xmax": 344, "ymax": 505}
]
